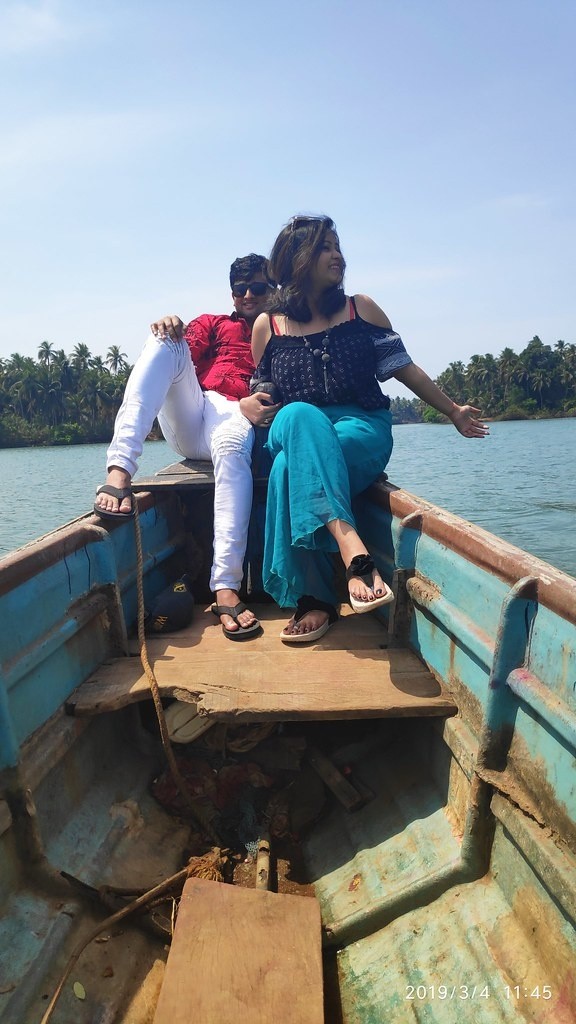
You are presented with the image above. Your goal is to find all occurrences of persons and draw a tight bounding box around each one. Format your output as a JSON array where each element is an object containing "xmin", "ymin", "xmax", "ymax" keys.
[
  {"xmin": 92, "ymin": 257, "xmax": 277, "ymax": 640},
  {"xmin": 239, "ymin": 213, "xmax": 491, "ymax": 642}
]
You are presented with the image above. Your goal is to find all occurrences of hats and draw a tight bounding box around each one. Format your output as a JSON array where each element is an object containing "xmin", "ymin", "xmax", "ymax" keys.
[{"xmin": 147, "ymin": 578, "xmax": 195, "ymax": 632}]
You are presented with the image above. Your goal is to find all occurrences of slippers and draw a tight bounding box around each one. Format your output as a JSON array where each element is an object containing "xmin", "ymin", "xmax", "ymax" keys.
[
  {"xmin": 94, "ymin": 485, "xmax": 136, "ymax": 522},
  {"xmin": 211, "ymin": 595, "xmax": 261, "ymax": 640},
  {"xmin": 345, "ymin": 554, "xmax": 395, "ymax": 614},
  {"xmin": 279, "ymin": 592, "xmax": 342, "ymax": 642}
]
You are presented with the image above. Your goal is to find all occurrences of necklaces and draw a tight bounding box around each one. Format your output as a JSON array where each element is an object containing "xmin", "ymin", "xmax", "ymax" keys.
[{"xmin": 295, "ymin": 317, "xmax": 332, "ymax": 394}]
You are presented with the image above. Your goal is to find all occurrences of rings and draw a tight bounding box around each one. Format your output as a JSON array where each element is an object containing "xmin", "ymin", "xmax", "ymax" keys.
[{"xmin": 264, "ymin": 419, "xmax": 269, "ymax": 424}]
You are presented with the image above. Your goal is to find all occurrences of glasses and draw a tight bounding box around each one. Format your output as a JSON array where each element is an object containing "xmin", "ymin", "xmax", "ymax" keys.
[
  {"xmin": 290, "ymin": 214, "xmax": 325, "ymax": 233},
  {"xmin": 233, "ymin": 282, "xmax": 269, "ymax": 298}
]
